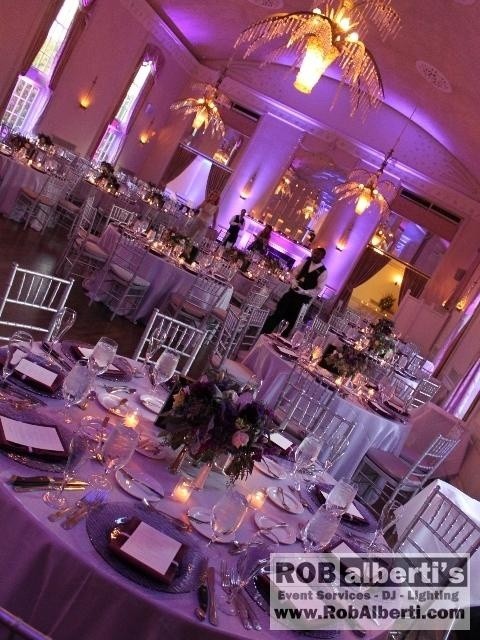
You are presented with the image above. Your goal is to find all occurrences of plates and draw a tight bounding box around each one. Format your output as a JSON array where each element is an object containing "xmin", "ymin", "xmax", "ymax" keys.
[
  {"xmin": 186, "ymin": 504, "xmax": 236, "ymax": 544},
  {"xmin": 315, "ymin": 483, "xmax": 368, "ymax": 525},
  {"xmin": 267, "ymin": 487, "xmax": 305, "ymax": 514},
  {"xmin": 105, "ymin": 512, "xmax": 183, "ymax": 580},
  {"xmin": 253, "ymin": 454, "xmax": 287, "ymax": 480},
  {"xmin": 254, "ymin": 510, "xmax": 297, "ymax": 545}
]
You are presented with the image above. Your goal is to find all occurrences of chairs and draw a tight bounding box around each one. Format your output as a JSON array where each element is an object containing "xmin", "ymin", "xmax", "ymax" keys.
[{"xmin": 1, "ymin": 118, "xmax": 480, "ymax": 639}]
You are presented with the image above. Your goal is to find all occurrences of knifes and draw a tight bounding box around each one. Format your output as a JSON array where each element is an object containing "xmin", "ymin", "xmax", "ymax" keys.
[
  {"xmin": 194, "ymin": 558, "xmax": 209, "ymax": 620},
  {"xmin": 208, "ymin": 562, "xmax": 220, "ymax": 627}
]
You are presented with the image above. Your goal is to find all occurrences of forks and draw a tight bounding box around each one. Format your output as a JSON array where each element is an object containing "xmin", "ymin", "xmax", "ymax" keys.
[
  {"xmin": 278, "ymin": 488, "xmax": 290, "ymax": 510},
  {"xmin": 217, "ymin": 559, "xmax": 263, "ymax": 633}
]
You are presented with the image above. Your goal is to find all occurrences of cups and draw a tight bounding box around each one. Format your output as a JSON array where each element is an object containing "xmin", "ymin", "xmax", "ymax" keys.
[
  {"xmin": 301, "ymin": 503, "xmax": 343, "ymax": 554},
  {"xmin": 244, "ymin": 486, "xmax": 269, "ymax": 509},
  {"xmin": 168, "ymin": 478, "xmax": 196, "ymax": 501},
  {"xmin": 1, "ymin": 330, "xmax": 188, "ymax": 533}
]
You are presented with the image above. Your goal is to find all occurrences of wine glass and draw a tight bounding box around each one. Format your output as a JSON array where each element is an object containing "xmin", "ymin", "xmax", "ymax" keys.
[
  {"xmin": 319, "ymin": 434, "xmax": 352, "ymax": 477},
  {"xmin": 41, "ymin": 306, "xmax": 77, "ymax": 348},
  {"xmin": 130, "ymin": 218, "xmax": 217, "ymax": 275},
  {"xmin": 360, "ymin": 499, "xmax": 405, "ymax": 552},
  {"xmin": 4, "ymin": 138, "xmax": 194, "ymax": 216},
  {"xmin": 204, "ymin": 498, "xmax": 246, "ymax": 551},
  {"xmin": 288, "ymin": 435, "xmax": 323, "ymax": 478},
  {"xmin": 246, "ymin": 247, "xmax": 289, "ymax": 281},
  {"xmin": 217, "ymin": 530, "xmax": 284, "ymax": 613},
  {"xmin": 321, "ymin": 479, "xmax": 358, "ymax": 522},
  {"xmin": 276, "ymin": 318, "xmax": 412, "ymax": 408}
]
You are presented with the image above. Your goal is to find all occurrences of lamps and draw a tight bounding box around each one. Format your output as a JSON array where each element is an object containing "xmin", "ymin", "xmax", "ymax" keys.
[
  {"xmin": 233, "ymin": 0, "xmax": 401, "ymax": 119},
  {"xmin": 331, "ymin": 57, "xmax": 455, "ymax": 220},
  {"xmin": 168, "ymin": 54, "xmax": 248, "ymax": 145},
  {"xmin": 76, "ymin": 74, "xmax": 99, "ymax": 111}
]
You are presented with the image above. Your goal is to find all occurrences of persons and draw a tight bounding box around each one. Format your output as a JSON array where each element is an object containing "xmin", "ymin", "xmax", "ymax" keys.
[
  {"xmin": 222, "ymin": 209, "xmax": 247, "ymax": 248},
  {"xmin": 259, "ymin": 247, "xmax": 327, "ymax": 335},
  {"xmin": 247, "ymin": 224, "xmax": 271, "ymax": 255},
  {"xmin": 182, "ymin": 189, "xmax": 220, "ymax": 242}
]
